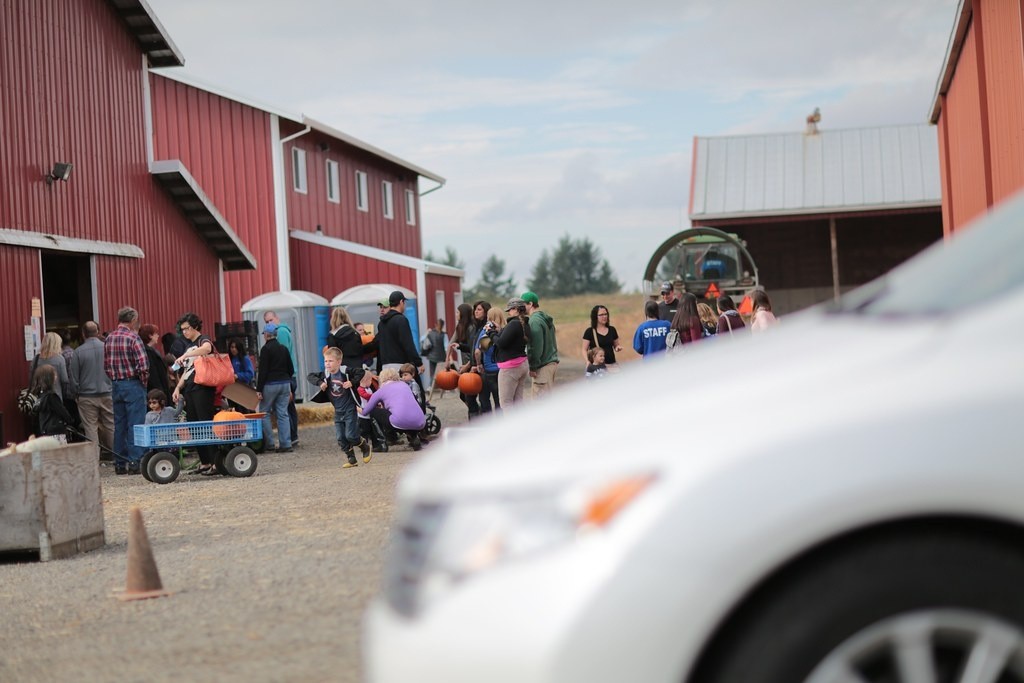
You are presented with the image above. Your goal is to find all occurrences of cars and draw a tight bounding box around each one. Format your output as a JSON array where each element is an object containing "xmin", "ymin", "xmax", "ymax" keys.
[{"xmin": 358, "ymin": 183, "xmax": 1024, "ymax": 682}]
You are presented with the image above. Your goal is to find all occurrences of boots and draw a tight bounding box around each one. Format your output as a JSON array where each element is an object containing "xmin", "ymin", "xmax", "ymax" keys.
[
  {"xmin": 357, "ymin": 438, "xmax": 372, "ymax": 463},
  {"xmin": 342, "ymin": 448, "xmax": 358, "ymax": 467}
]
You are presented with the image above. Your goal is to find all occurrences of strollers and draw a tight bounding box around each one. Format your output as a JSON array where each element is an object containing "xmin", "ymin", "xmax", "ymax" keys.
[{"xmin": 371, "ymin": 374, "xmax": 442, "ymax": 441}]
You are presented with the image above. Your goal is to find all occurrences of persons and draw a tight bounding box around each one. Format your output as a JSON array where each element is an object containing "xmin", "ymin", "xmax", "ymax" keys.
[
  {"xmin": 581, "ymin": 304, "xmax": 624, "ymax": 375},
  {"xmin": 28, "ymin": 307, "xmax": 227, "ymax": 476},
  {"xmin": 585, "ymin": 347, "xmax": 609, "ymax": 381},
  {"xmin": 227, "ymin": 337, "xmax": 255, "ymax": 415},
  {"xmin": 326, "ymin": 290, "xmax": 430, "ymax": 454},
  {"xmin": 306, "ymin": 348, "xmax": 372, "ymax": 467},
  {"xmin": 632, "ymin": 281, "xmax": 777, "ymax": 360},
  {"xmin": 418, "ymin": 317, "xmax": 450, "ymax": 395},
  {"xmin": 255, "ymin": 310, "xmax": 301, "ymax": 452},
  {"xmin": 444, "ymin": 291, "xmax": 560, "ymax": 422}
]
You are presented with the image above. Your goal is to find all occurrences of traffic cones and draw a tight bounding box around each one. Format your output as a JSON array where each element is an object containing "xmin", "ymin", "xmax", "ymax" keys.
[{"xmin": 113, "ymin": 507, "xmax": 184, "ymax": 602}]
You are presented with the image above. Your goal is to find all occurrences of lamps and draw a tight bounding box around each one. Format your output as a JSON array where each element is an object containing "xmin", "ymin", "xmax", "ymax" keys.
[{"xmin": 45, "ymin": 162, "xmax": 73, "ymax": 185}]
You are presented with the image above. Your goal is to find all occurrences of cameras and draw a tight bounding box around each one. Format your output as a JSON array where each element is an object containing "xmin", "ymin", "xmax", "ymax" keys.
[{"xmin": 483, "ymin": 321, "xmax": 495, "ymax": 330}]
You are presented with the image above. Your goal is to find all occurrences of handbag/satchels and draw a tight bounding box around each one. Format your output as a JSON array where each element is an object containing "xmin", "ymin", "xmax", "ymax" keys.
[
  {"xmin": 422, "ymin": 335, "xmax": 432, "ymax": 351},
  {"xmin": 194, "ymin": 338, "xmax": 236, "ymax": 385},
  {"xmin": 18, "ymin": 389, "xmax": 38, "ymax": 416}
]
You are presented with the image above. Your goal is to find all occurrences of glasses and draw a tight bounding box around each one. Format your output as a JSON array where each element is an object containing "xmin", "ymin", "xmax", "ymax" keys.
[
  {"xmin": 180, "ymin": 324, "xmax": 192, "ymax": 332},
  {"xmin": 598, "ymin": 312, "xmax": 608, "ymax": 317},
  {"xmin": 148, "ymin": 401, "xmax": 159, "ymax": 405},
  {"xmin": 662, "ymin": 292, "xmax": 671, "ymax": 296}
]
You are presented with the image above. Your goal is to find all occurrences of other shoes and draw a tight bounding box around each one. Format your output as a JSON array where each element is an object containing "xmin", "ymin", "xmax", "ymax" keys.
[
  {"xmin": 276, "ymin": 445, "xmax": 292, "ymax": 452},
  {"xmin": 128, "ymin": 463, "xmax": 141, "ymax": 475},
  {"xmin": 385, "ymin": 438, "xmax": 405, "ymax": 446},
  {"xmin": 373, "ymin": 442, "xmax": 389, "ymax": 453},
  {"xmin": 291, "ymin": 437, "xmax": 299, "ymax": 445},
  {"xmin": 115, "ymin": 465, "xmax": 128, "ymax": 475},
  {"xmin": 188, "ymin": 463, "xmax": 211, "ymax": 474},
  {"xmin": 264, "ymin": 447, "xmax": 274, "ymax": 452},
  {"xmin": 201, "ymin": 466, "xmax": 220, "ymax": 476},
  {"xmin": 413, "ymin": 444, "xmax": 422, "ymax": 451}
]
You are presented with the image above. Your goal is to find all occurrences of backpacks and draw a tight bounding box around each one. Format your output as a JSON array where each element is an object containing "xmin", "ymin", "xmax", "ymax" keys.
[{"xmin": 664, "ymin": 316, "xmax": 682, "ymax": 352}]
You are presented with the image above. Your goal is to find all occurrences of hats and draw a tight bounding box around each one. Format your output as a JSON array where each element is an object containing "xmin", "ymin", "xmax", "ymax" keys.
[
  {"xmin": 377, "ymin": 298, "xmax": 390, "ymax": 308},
  {"xmin": 521, "ymin": 291, "xmax": 538, "ymax": 305},
  {"xmin": 661, "ymin": 281, "xmax": 673, "ymax": 292},
  {"xmin": 389, "ymin": 291, "xmax": 408, "ymax": 305},
  {"xmin": 261, "ymin": 323, "xmax": 278, "ymax": 334},
  {"xmin": 504, "ymin": 298, "xmax": 525, "ymax": 312}
]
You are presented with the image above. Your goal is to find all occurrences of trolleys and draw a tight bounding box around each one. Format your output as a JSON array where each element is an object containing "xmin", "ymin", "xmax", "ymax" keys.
[{"xmin": 61, "ymin": 416, "xmax": 264, "ymax": 485}]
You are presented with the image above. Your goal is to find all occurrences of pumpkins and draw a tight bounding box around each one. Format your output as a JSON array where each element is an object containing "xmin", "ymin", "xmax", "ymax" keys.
[
  {"xmin": 361, "ymin": 331, "xmax": 378, "ymax": 357},
  {"xmin": 436, "ymin": 367, "xmax": 461, "ymax": 390},
  {"xmin": 322, "ymin": 345, "xmax": 329, "ymax": 353},
  {"xmin": 458, "ymin": 370, "xmax": 482, "ymax": 395},
  {"xmin": 212, "ymin": 408, "xmax": 246, "ymax": 439}
]
